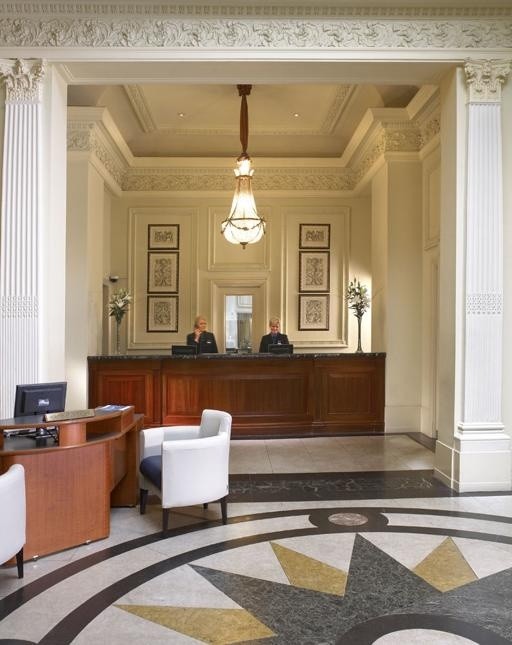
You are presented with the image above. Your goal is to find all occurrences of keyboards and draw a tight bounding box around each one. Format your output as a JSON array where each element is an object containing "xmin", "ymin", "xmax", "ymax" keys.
[{"xmin": 4, "ymin": 428, "xmax": 37, "ymax": 438}]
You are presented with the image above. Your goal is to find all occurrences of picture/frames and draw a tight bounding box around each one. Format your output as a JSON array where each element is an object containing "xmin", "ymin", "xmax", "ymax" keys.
[
  {"xmin": 147, "ymin": 223, "xmax": 180, "ymax": 250},
  {"xmin": 298, "ymin": 250, "xmax": 331, "ymax": 292},
  {"xmin": 297, "ymin": 293, "xmax": 331, "ymax": 332},
  {"xmin": 299, "ymin": 222, "xmax": 331, "ymax": 249},
  {"xmin": 146, "ymin": 295, "xmax": 180, "ymax": 333},
  {"xmin": 147, "ymin": 251, "xmax": 180, "ymax": 294}
]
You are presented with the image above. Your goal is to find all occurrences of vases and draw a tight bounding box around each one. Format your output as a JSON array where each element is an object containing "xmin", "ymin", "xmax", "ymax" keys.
[
  {"xmin": 114, "ymin": 318, "xmax": 124, "ymax": 357},
  {"xmin": 355, "ymin": 318, "xmax": 365, "ymax": 353}
]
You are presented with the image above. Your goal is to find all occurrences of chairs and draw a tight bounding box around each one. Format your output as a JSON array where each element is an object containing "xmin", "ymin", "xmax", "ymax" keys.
[
  {"xmin": 1, "ymin": 462, "xmax": 28, "ymax": 583},
  {"xmin": 138, "ymin": 408, "xmax": 233, "ymax": 538}
]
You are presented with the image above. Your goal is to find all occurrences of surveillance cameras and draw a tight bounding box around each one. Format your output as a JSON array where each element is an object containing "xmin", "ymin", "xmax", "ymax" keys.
[{"xmin": 109, "ymin": 275, "xmax": 119, "ymax": 283}]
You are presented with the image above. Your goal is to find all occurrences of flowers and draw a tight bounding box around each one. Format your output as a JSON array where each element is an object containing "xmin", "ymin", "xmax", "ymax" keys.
[
  {"xmin": 108, "ymin": 285, "xmax": 134, "ymax": 322},
  {"xmin": 344, "ymin": 276, "xmax": 372, "ymax": 317}
]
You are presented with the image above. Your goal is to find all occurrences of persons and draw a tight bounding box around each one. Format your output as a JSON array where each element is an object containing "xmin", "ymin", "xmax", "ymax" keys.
[
  {"xmin": 187, "ymin": 315, "xmax": 219, "ymax": 354},
  {"xmin": 259, "ymin": 316, "xmax": 289, "ymax": 353}
]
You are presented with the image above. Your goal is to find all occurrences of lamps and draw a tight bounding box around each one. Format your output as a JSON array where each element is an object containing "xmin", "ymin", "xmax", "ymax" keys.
[{"xmin": 219, "ymin": 83, "xmax": 270, "ymax": 250}]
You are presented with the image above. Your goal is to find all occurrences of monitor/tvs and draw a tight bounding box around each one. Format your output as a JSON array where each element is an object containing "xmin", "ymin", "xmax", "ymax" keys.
[
  {"xmin": 267, "ymin": 344, "xmax": 293, "ymax": 354},
  {"xmin": 171, "ymin": 344, "xmax": 198, "ymax": 355},
  {"xmin": 13, "ymin": 381, "xmax": 68, "ymax": 417}
]
